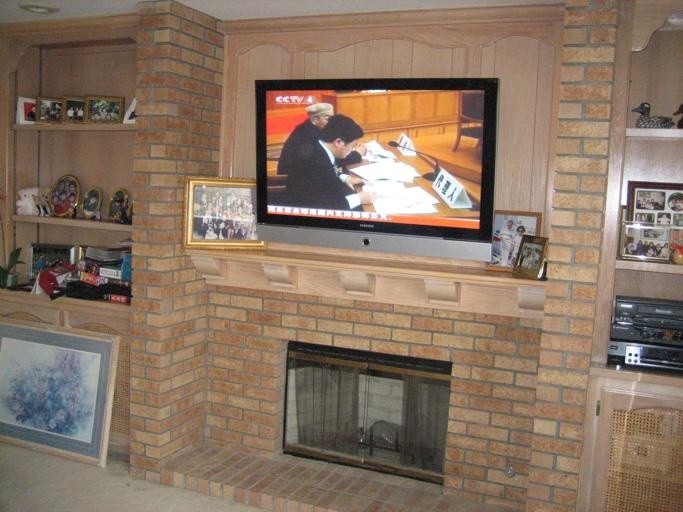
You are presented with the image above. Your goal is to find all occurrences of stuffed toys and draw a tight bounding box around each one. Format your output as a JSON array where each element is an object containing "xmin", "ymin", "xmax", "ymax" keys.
[{"xmin": 16, "ymin": 186, "xmax": 52, "ymax": 217}]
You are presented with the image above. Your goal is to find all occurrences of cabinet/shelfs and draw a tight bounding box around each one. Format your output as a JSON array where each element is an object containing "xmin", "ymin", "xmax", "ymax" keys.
[
  {"xmin": 575, "ymin": 1, "xmax": 682, "ymax": 511},
  {"xmin": 0, "ymin": 37, "xmax": 137, "ymax": 464}
]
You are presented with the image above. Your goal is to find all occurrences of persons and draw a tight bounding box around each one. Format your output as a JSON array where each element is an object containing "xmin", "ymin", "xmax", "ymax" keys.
[
  {"xmin": 53, "ymin": 180, "xmax": 122, "ymax": 217},
  {"xmin": 194, "ymin": 191, "xmax": 257, "ymax": 240},
  {"xmin": 28, "ymin": 105, "xmax": 111, "ymax": 121},
  {"xmin": 277, "ymin": 102, "xmax": 365, "ymax": 175},
  {"xmin": 286, "ymin": 115, "xmax": 376, "ymax": 211},
  {"xmin": 36, "ymin": 255, "xmax": 69, "ymax": 273},
  {"xmin": 624, "ymin": 198, "xmax": 683, "ymax": 258},
  {"xmin": 499, "ymin": 220, "xmax": 543, "ymax": 271}
]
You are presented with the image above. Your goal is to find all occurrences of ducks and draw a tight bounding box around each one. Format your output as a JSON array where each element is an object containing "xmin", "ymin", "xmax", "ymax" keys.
[{"xmin": 630, "ymin": 102, "xmax": 674, "ymax": 129}]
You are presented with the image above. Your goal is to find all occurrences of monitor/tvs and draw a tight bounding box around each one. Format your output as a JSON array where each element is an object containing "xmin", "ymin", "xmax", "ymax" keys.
[{"xmin": 255, "ymin": 77, "xmax": 497, "ymax": 263}]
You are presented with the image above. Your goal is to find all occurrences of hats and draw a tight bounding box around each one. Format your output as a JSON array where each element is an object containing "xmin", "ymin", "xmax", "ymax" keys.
[{"xmin": 306, "ymin": 103, "xmax": 333, "ymax": 117}]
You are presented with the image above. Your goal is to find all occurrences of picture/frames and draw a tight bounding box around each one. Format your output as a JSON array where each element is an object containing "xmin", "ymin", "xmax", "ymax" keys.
[
  {"xmin": 484, "ymin": 208, "xmax": 543, "ymax": 273},
  {"xmin": 0, "ymin": 318, "xmax": 120, "ymax": 469},
  {"xmin": 47, "ymin": 174, "xmax": 129, "ymax": 222},
  {"xmin": 615, "ymin": 179, "xmax": 683, "ymax": 265},
  {"xmin": 27, "ymin": 241, "xmax": 77, "ymax": 282},
  {"xmin": 13, "ymin": 94, "xmax": 125, "ymax": 124},
  {"xmin": 181, "ymin": 174, "xmax": 267, "ymax": 252},
  {"xmin": 511, "ymin": 232, "xmax": 549, "ymax": 280}
]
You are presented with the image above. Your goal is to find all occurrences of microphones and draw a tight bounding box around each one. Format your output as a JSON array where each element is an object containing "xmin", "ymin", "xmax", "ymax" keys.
[{"xmin": 388, "ymin": 140, "xmax": 439, "ymax": 181}]
